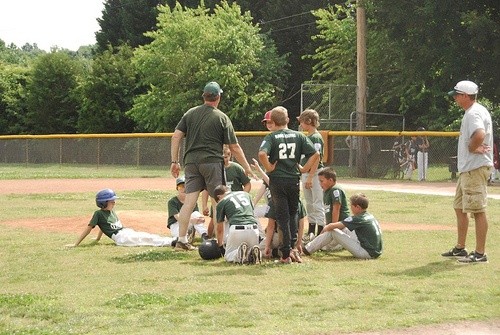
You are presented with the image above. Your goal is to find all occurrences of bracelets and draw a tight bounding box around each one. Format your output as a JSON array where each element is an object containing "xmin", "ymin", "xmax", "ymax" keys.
[
  {"xmin": 171, "ymin": 160, "xmax": 180, "ymax": 164},
  {"xmin": 171, "ymin": 161, "xmax": 179, "ymax": 164}
]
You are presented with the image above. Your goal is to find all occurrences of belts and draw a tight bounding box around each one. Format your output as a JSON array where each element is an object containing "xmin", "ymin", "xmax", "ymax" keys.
[{"xmin": 235, "ymin": 225, "xmax": 257, "ymax": 229}]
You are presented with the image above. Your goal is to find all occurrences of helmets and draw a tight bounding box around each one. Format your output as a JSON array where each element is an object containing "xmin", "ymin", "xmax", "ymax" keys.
[
  {"xmin": 96, "ymin": 189, "xmax": 116, "ymax": 201},
  {"xmin": 261, "ymin": 111, "xmax": 271, "ymax": 123},
  {"xmin": 198, "ymin": 239, "xmax": 222, "ymax": 259}
]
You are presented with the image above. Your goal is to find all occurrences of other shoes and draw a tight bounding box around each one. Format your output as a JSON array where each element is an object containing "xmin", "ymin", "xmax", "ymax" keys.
[
  {"xmin": 278, "ymin": 248, "xmax": 303, "ymax": 264},
  {"xmin": 441, "ymin": 248, "xmax": 468, "ymax": 257},
  {"xmin": 457, "ymin": 252, "xmax": 488, "ymax": 263},
  {"xmin": 251, "ymin": 245, "xmax": 262, "ymax": 265},
  {"xmin": 175, "ymin": 241, "xmax": 198, "ymax": 252},
  {"xmin": 239, "ymin": 242, "xmax": 249, "ymax": 266}
]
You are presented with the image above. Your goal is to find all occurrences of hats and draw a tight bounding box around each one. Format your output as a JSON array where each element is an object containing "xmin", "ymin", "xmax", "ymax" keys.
[
  {"xmin": 204, "ymin": 82, "xmax": 223, "ymax": 94},
  {"xmin": 176, "ymin": 177, "xmax": 185, "ymax": 185},
  {"xmin": 447, "ymin": 80, "xmax": 478, "ymax": 97}
]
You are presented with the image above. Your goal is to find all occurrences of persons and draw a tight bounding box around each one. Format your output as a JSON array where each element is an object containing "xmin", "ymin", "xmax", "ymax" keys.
[
  {"xmin": 345, "ymin": 136, "xmax": 357, "ymax": 166},
  {"xmin": 318, "ymin": 167, "xmax": 350, "ymax": 251},
  {"xmin": 169, "ymin": 82, "xmax": 259, "ymax": 251},
  {"xmin": 65, "ymin": 189, "xmax": 193, "ymax": 247},
  {"xmin": 251, "ymin": 106, "xmax": 324, "ymax": 264},
  {"xmin": 441, "ymin": 80, "xmax": 493, "ymax": 264},
  {"xmin": 392, "ymin": 142, "xmax": 402, "ymax": 179},
  {"xmin": 302, "ymin": 194, "xmax": 383, "ymax": 259},
  {"xmin": 214, "ymin": 185, "xmax": 261, "ymax": 265},
  {"xmin": 202, "ymin": 144, "xmax": 251, "ymax": 238},
  {"xmin": 167, "ymin": 178, "xmax": 212, "ymax": 242},
  {"xmin": 404, "ymin": 136, "xmax": 417, "ymax": 180},
  {"xmin": 416, "ymin": 127, "xmax": 430, "ymax": 181}
]
